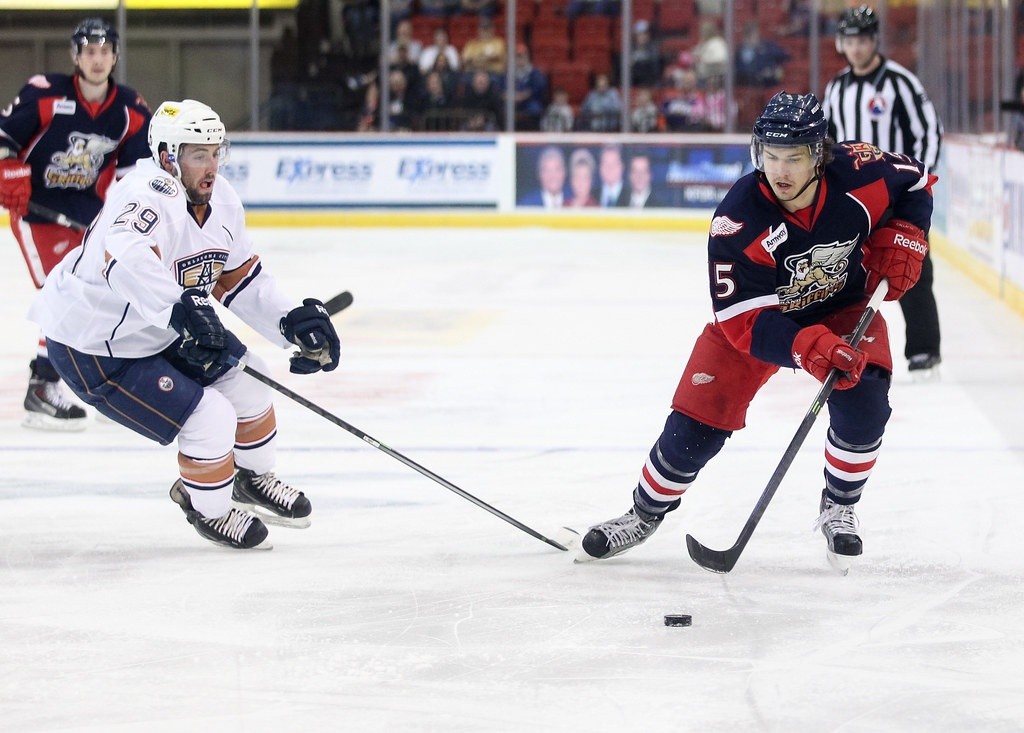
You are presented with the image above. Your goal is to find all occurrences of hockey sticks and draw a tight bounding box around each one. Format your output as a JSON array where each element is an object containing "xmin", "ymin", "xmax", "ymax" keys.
[
  {"xmin": 685, "ymin": 276, "xmax": 888, "ymax": 577},
  {"xmin": 226, "ymin": 354, "xmax": 582, "ymax": 553},
  {"xmin": 24, "ymin": 201, "xmax": 88, "ymax": 234}
]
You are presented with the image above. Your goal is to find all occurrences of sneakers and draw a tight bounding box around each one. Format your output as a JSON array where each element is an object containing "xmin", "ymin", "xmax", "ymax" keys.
[
  {"xmin": 23, "ymin": 359, "xmax": 87, "ymax": 432},
  {"xmin": 907, "ymin": 350, "xmax": 942, "ymax": 378},
  {"xmin": 573, "ymin": 489, "xmax": 682, "ymax": 562},
  {"xmin": 170, "ymin": 479, "xmax": 274, "ymax": 550},
  {"xmin": 231, "ymin": 462, "xmax": 312, "ymax": 528},
  {"xmin": 813, "ymin": 490, "xmax": 864, "ymax": 575}
]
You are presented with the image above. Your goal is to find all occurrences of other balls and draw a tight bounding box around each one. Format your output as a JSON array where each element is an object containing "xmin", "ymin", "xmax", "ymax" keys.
[{"xmin": 663, "ymin": 614, "xmax": 694, "ymax": 628}]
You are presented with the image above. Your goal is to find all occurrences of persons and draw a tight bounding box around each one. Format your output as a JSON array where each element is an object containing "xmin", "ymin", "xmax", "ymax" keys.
[
  {"xmin": 0, "ymin": 17, "xmax": 151, "ymax": 437},
  {"xmin": 735, "ymin": 23, "xmax": 792, "ymax": 86},
  {"xmin": 565, "ymin": 1, "xmax": 851, "ymax": 38},
  {"xmin": 38, "ymin": 100, "xmax": 341, "ymax": 550},
  {"xmin": 616, "ymin": 151, "xmax": 663, "ymax": 207},
  {"xmin": 564, "ymin": 148, "xmax": 599, "ymax": 207},
  {"xmin": 573, "ymin": 95, "xmax": 932, "ymax": 572},
  {"xmin": 515, "ymin": 145, "xmax": 574, "ymax": 207},
  {"xmin": 690, "ymin": 75, "xmax": 740, "ymax": 131},
  {"xmin": 818, "ymin": 2, "xmax": 946, "ymax": 383},
  {"xmin": 581, "ymin": 76, "xmax": 624, "ymax": 130},
  {"xmin": 689, "ymin": 21, "xmax": 729, "ymax": 85},
  {"xmin": 629, "ymin": 92, "xmax": 660, "ymax": 133},
  {"xmin": 624, "ymin": 23, "xmax": 669, "ymax": 90},
  {"xmin": 271, "ymin": 1, "xmax": 552, "ymax": 132},
  {"xmin": 540, "ymin": 89, "xmax": 572, "ymax": 132},
  {"xmin": 593, "ymin": 144, "xmax": 631, "ymax": 207}
]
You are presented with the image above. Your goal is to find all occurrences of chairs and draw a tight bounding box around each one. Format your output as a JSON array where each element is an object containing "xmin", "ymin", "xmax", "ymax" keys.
[{"xmin": 395, "ymin": 0, "xmax": 1023, "ymax": 134}]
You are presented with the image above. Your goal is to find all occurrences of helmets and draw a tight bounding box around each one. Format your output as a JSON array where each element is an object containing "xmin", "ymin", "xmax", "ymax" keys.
[
  {"xmin": 751, "ymin": 91, "xmax": 828, "ymax": 174},
  {"xmin": 148, "ymin": 98, "xmax": 225, "ymax": 177},
  {"xmin": 833, "ymin": 6, "xmax": 879, "ymax": 51},
  {"xmin": 70, "ymin": 17, "xmax": 120, "ymax": 59}
]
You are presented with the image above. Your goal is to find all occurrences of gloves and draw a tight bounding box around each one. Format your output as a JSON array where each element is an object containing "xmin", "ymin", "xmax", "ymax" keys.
[
  {"xmin": 279, "ymin": 297, "xmax": 341, "ymax": 374},
  {"xmin": 171, "ymin": 288, "xmax": 227, "ymax": 366},
  {"xmin": 860, "ymin": 220, "xmax": 929, "ymax": 300},
  {"xmin": 791, "ymin": 325, "xmax": 869, "ymax": 391},
  {"xmin": 0, "ymin": 161, "xmax": 33, "ymax": 218}
]
